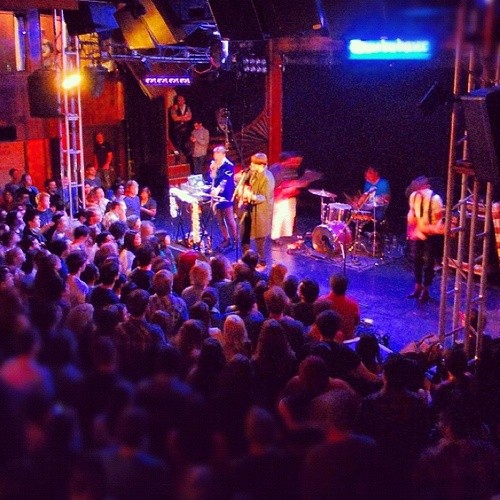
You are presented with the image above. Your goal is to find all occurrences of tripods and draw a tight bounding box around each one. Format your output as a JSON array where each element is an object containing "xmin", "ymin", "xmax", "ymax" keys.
[{"xmin": 346, "ymin": 217, "xmax": 370, "ymax": 259}]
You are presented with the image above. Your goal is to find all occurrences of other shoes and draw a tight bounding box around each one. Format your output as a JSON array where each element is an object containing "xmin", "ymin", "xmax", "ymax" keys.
[
  {"xmin": 408, "ymin": 290, "xmax": 420, "ymax": 297},
  {"xmin": 419, "ymin": 290, "xmax": 428, "ymax": 303},
  {"xmin": 234, "ymin": 239, "xmax": 240, "ymax": 249},
  {"xmin": 219, "ymin": 237, "xmax": 231, "ymax": 248},
  {"xmin": 256, "ymin": 264, "xmax": 267, "ymax": 272}
]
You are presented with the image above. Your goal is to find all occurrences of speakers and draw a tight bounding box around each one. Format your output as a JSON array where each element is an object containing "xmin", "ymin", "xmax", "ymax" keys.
[
  {"xmin": 461, "ymin": 84, "xmax": 500, "ymax": 182},
  {"xmin": 27, "ymin": 67, "xmax": 65, "ymax": 119}
]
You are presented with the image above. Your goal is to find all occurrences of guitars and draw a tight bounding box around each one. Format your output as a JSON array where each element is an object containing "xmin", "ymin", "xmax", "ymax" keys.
[{"xmin": 408, "ymin": 217, "xmax": 447, "ymax": 240}]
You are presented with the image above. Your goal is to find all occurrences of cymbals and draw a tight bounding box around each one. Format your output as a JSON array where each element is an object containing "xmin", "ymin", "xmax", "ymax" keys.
[
  {"xmin": 364, "ymin": 201, "xmax": 384, "ymax": 207},
  {"xmin": 308, "ymin": 189, "xmax": 337, "ymax": 198}
]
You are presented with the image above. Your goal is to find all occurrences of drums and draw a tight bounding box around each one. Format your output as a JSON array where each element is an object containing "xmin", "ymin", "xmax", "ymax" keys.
[
  {"xmin": 326, "ymin": 203, "xmax": 352, "ymax": 223},
  {"xmin": 350, "ymin": 210, "xmax": 372, "ymax": 220},
  {"xmin": 312, "ymin": 220, "xmax": 353, "ymax": 255}
]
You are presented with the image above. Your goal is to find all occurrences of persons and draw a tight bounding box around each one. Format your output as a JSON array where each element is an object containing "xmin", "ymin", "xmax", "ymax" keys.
[
  {"xmin": 203, "ymin": 146, "xmax": 242, "ymax": 250},
  {"xmin": 188, "ymin": 119, "xmax": 210, "ymax": 174},
  {"xmin": 0, "ymin": 130, "xmax": 500, "ymax": 500},
  {"xmin": 351, "ymin": 164, "xmax": 391, "ymax": 240},
  {"xmin": 169, "ymin": 95, "xmax": 194, "ymax": 163},
  {"xmin": 235, "ymin": 153, "xmax": 276, "ymax": 273},
  {"xmin": 405, "ymin": 175, "xmax": 444, "ymax": 302},
  {"xmin": 269, "ymin": 150, "xmax": 322, "ymax": 247}
]
